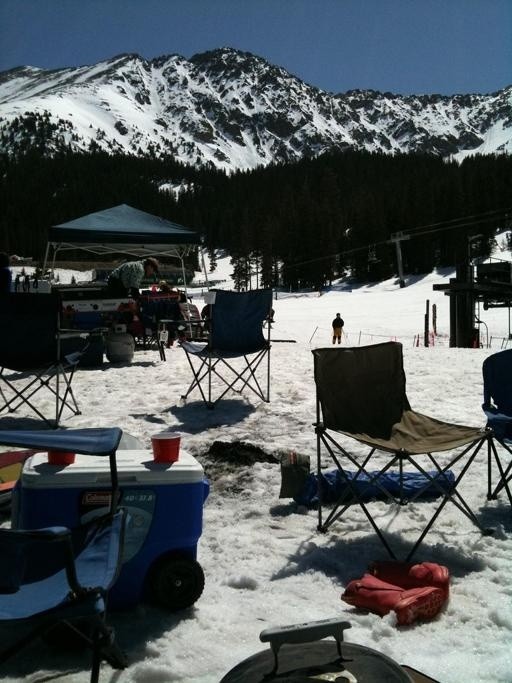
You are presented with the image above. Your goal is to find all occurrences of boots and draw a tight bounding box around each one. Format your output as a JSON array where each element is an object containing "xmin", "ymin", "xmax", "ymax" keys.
[{"xmin": 341, "ymin": 559, "xmax": 450, "ymax": 626}]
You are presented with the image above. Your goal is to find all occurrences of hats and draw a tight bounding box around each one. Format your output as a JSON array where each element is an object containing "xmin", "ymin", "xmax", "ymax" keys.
[{"xmin": 147, "ymin": 257, "xmax": 159, "ymax": 273}]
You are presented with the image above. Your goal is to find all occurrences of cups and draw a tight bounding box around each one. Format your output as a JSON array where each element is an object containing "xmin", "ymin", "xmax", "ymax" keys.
[{"xmin": 48, "ymin": 446, "xmax": 76, "ymax": 465}]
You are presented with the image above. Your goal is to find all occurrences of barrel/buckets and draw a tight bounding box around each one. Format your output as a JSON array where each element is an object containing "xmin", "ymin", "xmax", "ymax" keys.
[{"xmin": 47, "ymin": 451, "xmax": 75, "ymax": 466}]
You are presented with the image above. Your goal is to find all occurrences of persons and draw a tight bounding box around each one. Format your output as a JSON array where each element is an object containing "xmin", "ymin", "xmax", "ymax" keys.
[
  {"xmin": 158, "ymin": 280, "xmax": 169, "ymax": 292},
  {"xmin": 106, "ymin": 257, "xmax": 159, "ymax": 298},
  {"xmin": 332, "ymin": 313, "xmax": 344, "ymax": 344}
]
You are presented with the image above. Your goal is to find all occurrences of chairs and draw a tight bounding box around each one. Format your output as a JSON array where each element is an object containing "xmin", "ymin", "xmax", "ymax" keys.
[
  {"xmin": 2, "ymin": 287, "xmax": 84, "ymax": 422},
  {"xmin": 0, "ymin": 424, "xmax": 134, "ymax": 682},
  {"xmin": 136, "ymin": 298, "xmax": 171, "ymax": 362},
  {"xmin": 171, "ymin": 284, "xmax": 276, "ymax": 406},
  {"xmin": 304, "ymin": 339, "xmax": 495, "ymax": 566},
  {"xmin": 475, "ymin": 343, "xmax": 512, "ymax": 506}
]
